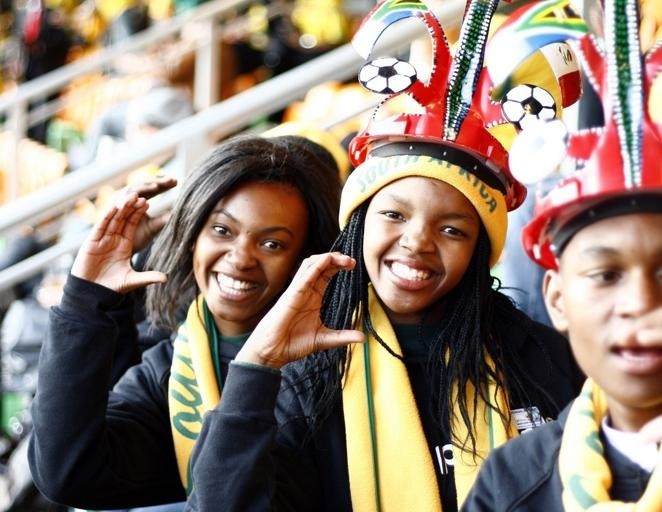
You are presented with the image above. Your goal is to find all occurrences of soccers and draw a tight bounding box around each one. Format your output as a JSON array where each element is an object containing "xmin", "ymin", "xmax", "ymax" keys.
[
  {"xmin": 358, "ymin": 56, "xmax": 416, "ymax": 95},
  {"xmin": 503, "ymin": 84, "xmax": 556, "ymax": 129}
]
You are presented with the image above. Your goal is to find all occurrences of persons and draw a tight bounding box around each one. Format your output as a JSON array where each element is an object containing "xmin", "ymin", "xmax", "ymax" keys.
[
  {"xmin": 28, "ymin": 135, "xmax": 342, "ymax": 512},
  {"xmin": 1, "ymin": 330, "xmax": 69, "ymax": 512},
  {"xmin": 451, "ymin": 187, "xmax": 662, "ymax": 512},
  {"xmin": 0, "ymin": 0, "xmax": 587, "ymax": 330},
  {"xmin": 182, "ymin": 141, "xmax": 589, "ymax": 511}
]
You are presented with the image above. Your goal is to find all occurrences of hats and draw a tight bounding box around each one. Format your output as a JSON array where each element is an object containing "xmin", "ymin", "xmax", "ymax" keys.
[
  {"xmin": 338, "ymin": 155, "xmax": 508, "ymax": 269},
  {"xmin": 351, "ymin": 101, "xmax": 527, "ymax": 212},
  {"xmin": 523, "ymin": 112, "xmax": 662, "ymax": 271}
]
